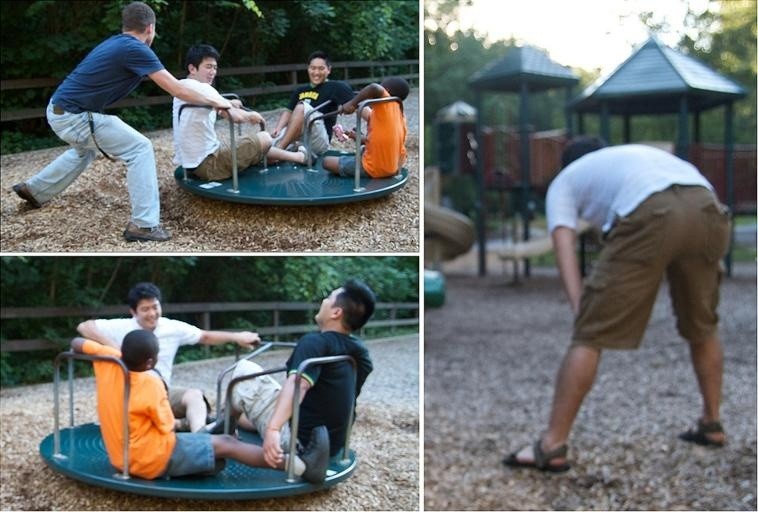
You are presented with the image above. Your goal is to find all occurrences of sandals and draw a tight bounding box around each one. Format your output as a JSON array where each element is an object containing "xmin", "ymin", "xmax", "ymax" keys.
[
  {"xmin": 677, "ymin": 419, "xmax": 725, "ymax": 446},
  {"xmin": 502, "ymin": 439, "xmax": 570, "ymax": 471}
]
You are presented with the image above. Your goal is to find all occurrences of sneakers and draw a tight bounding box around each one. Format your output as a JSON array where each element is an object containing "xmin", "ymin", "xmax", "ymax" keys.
[
  {"xmin": 124, "ymin": 223, "xmax": 169, "ymax": 241},
  {"xmin": 181, "ymin": 415, "xmax": 234, "ymax": 476},
  {"xmin": 12, "ymin": 182, "xmax": 42, "ymax": 208},
  {"xmin": 297, "ymin": 425, "xmax": 330, "ymax": 485},
  {"xmin": 259, "ymin": 143, "xmax": 318, "ymax": 167}
]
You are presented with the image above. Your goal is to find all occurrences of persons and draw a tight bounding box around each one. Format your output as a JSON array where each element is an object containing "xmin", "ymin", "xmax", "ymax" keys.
[
  {"xmin": 322, "ymin": 75, "xmax": 409, "ymax": 178},
  {"xmin": 71, "ymin": 328, "xmax": 331, "ymax": 485},
  {"xmin": 76, "ymin": 281, "xmax": 262, "ymax": 433},
  {"xmin": 12, "ymin": 3, "xmax": 233, "ymax": 241},
  {"xmin": 503, "ymin": 133, "xmax": 729, "ymax": 469},
  {"xmin": 229, "ymin": 279, "xmax": 373, "ymax": 467},
  {"xmin": 273, "ymin": 53, "xmax": 370, "ymax": 153},
  {"xmin": 172, "ymin": 45, "xmax": 315, "ymax": 180}
]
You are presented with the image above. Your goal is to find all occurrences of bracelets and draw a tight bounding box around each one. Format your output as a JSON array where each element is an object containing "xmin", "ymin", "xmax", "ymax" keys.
[{"xmin": 265, "ymin": 426, "xmax": 280, "ymax": 432}]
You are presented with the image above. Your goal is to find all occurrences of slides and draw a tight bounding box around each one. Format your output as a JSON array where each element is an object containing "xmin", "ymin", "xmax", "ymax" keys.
[{"xmin": 423, "ymin": 165, "xmax": 476, "ymax": 268}]
